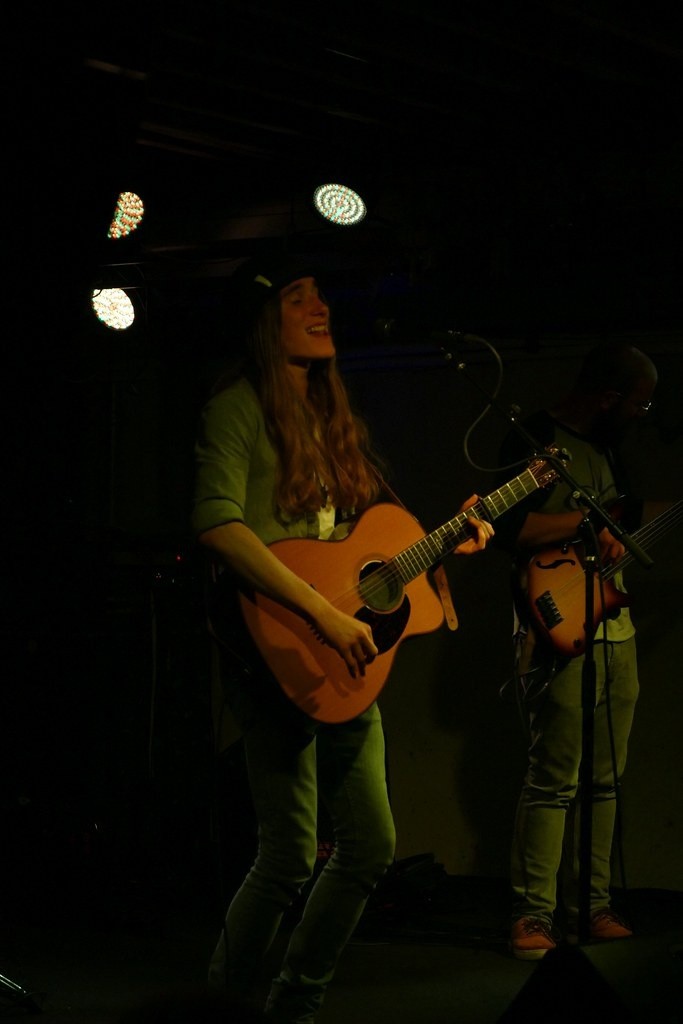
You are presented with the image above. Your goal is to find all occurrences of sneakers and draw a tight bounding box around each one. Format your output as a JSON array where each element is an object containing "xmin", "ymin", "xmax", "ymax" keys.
[
  {"xmin": 504, "ymin": 916, "xmax": 557, "ymax": 962},
  {"xmin": 590, "ymin": 909, "xmax": 633, "ymax": 940}
]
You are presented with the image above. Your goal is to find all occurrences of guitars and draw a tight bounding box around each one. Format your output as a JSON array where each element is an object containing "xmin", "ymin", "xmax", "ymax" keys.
[
  {"xmin": 511, "ymin": 493, "xmax": 682, "ymax": 657},
  {"xmin": 211, "ymin": 442, "xmax": 572, "ymax": 725}
]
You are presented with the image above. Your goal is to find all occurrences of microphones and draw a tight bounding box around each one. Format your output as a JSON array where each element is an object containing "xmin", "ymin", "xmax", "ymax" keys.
[{"xmin": 374, "ymin": 317, "xmax": 470, "ymax": 347}]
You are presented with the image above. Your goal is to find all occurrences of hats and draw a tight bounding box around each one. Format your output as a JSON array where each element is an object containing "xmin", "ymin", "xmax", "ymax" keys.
[{"xmin": 210, "ymin": 237, "xmax": 314, "ymax": 337}]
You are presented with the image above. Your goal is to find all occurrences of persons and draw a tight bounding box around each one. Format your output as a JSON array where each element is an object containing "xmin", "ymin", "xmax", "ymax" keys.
[
  {"xmin": 191, "ymin": 258, "xmax": 494, "ymax": 1024},
  {"xmin": 461, "ymin": 346, "xmax": 683, "ymax": 960}
]
